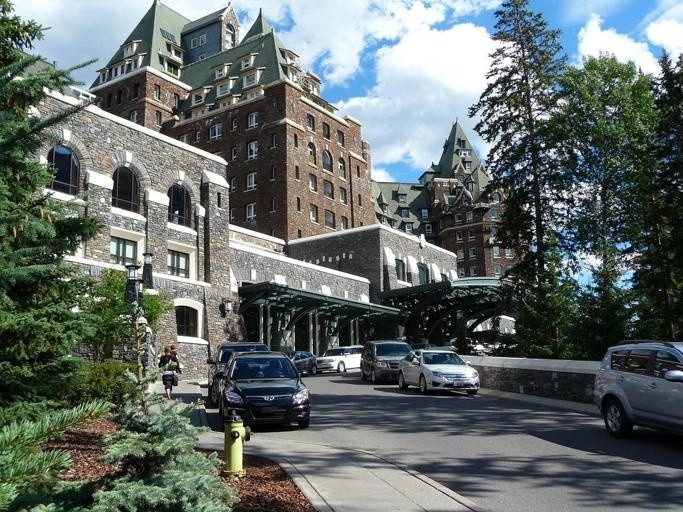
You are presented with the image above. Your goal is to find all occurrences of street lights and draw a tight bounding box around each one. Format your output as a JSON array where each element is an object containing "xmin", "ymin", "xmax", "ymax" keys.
[{"xmin": 125, "ymin": 264, "xmax": 144, "ymax": 400}]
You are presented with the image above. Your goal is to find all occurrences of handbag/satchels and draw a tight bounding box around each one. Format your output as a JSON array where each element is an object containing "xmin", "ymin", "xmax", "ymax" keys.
[{"xmin": 173, "ymin": 375, "xmax": 178, "ymax": 386}]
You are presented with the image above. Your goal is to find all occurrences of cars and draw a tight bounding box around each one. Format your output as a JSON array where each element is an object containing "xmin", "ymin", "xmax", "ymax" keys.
[
  {"xmin": 207, "ymin": 340, "xmax": 480, "ymax": 429},
  {"xmin": 592, "ymin": 339, "xmax": 683, "ymax": 438}
]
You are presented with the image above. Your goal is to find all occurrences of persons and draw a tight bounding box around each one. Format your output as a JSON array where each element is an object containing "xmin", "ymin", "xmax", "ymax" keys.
[{"xmin": 159, "ymin": 345, "xmax": 182, "ymax": 400}]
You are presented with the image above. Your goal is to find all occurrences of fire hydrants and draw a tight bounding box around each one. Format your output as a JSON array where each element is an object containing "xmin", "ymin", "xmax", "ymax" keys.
[{"xmin": 224, "ymin": 408, "xmax": 253, "ymax": 479}]
